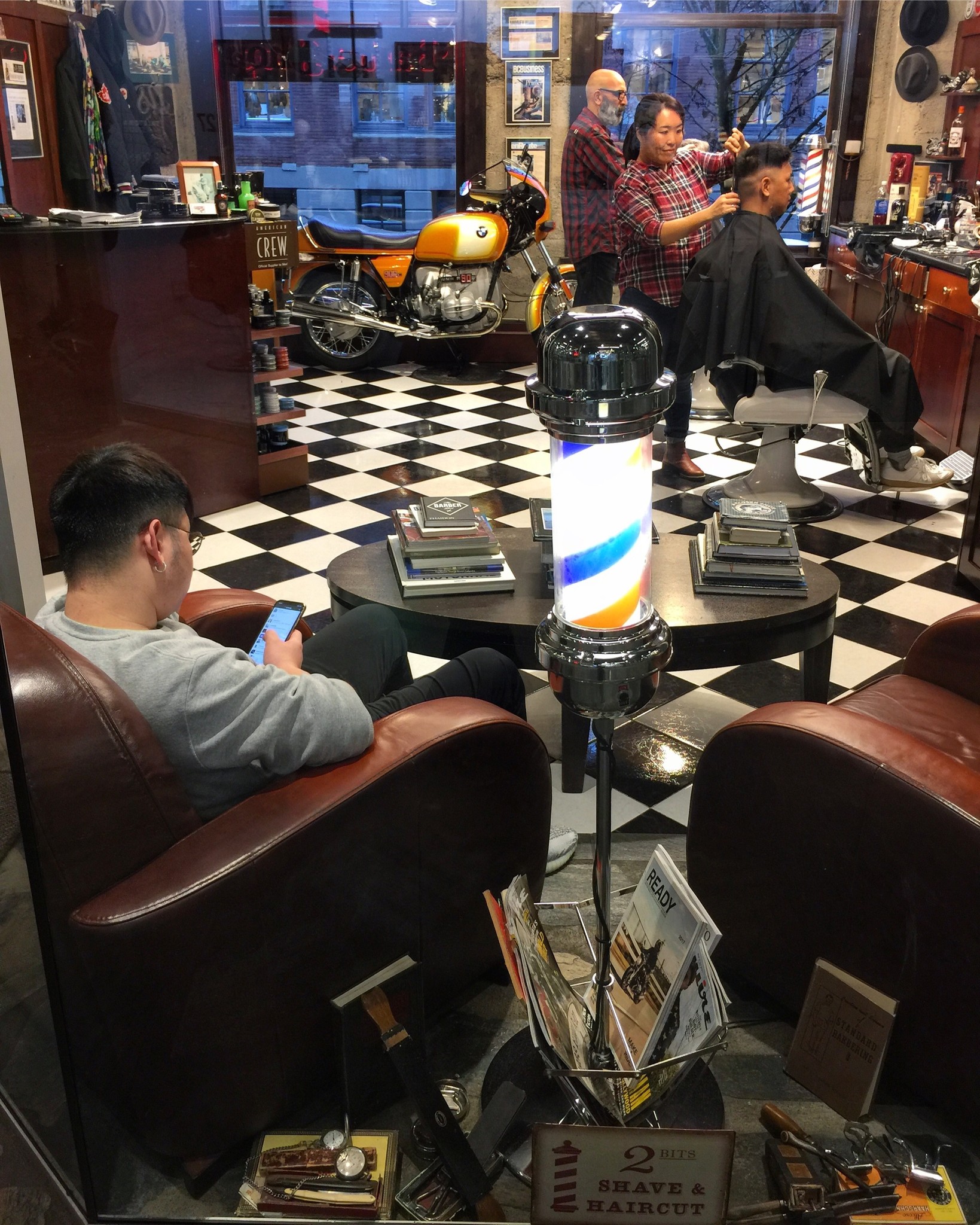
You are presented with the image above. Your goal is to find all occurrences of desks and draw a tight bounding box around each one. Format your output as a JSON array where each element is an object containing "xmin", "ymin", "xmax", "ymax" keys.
[
  {"xmin": 1, "ymin": 214, "xmax": 262, "ymax": 578},
  {"xmin": 328, "ymin": 527, "xmax": 841, "ymax": 794}
]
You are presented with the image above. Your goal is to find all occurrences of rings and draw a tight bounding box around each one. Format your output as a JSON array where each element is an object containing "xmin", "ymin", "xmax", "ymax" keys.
[{"xmin": 731, "ymin": 204, "xmax": 734, "ymax": 208}]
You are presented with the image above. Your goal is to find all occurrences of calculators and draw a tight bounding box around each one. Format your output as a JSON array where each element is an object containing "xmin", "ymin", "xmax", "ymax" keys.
[{"xmin": 0, "ymin": 203, "xmax": 24, "ymax": 224}]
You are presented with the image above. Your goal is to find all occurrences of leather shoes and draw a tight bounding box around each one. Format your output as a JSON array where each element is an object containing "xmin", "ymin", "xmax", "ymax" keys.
[{"xmin": 661, "ymin": 442, "xmax": 706, "ymax": 481}]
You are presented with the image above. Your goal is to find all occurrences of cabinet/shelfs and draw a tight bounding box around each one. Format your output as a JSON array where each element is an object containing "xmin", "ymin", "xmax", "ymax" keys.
[
  {"xmin": 250, "ymin": 318, "xmax": 311, "ymax": 497},
  {"xmin": 882, "ymin": 234, "xmax": 980, "ymax": 462},
  {"xmin": 825, "ymin": 223, "xmax": 917, "ymax": 341},
  {"xmin": 924, "ymin": 89, "xmax": 980, "ymax": 162}
]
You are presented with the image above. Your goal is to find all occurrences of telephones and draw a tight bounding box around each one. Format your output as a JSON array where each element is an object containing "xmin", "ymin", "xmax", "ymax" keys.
[{"xmin": 886, "ymin": 152, "xmax": 915, "ymax": 194}]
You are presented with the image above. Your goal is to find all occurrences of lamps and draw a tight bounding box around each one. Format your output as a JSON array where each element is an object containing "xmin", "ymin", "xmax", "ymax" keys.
[
  {"xmin": 645, "ymin": 1, "xmax": 657, "ymax": 9},
  {"xmin": 599, "ymin": 1, "xmax": 622, "ymax": 15},
  {"xmin": 529, "ymin": 301, "xmax": 679, "ymax": 1065},
  {"xmin": 426, "ymin": 16, "xmax": 440, "ymax": 30},
  {"xmin": 797, "ymin": 134, "xmax": 832, "ymax": 233}
]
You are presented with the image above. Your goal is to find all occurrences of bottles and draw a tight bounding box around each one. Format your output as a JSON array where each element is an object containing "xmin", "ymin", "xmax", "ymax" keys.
[
  {"xmin": 941, "ymin": 218, "xmax": 951, "ymax": 243},
  {"xmin": 215, "ymin": 180, "xmax": 228, "ymax": 218},
  {"xmin": 238, "ymin": 172, "xmax": 255, "ymax": 218},
  {"xmin": 890, "ymin": 185, "xmax": 908, "ymax": 229},
  {"xmin": 261, "ymin": 290, "xmax": 275, "ymax": 316},
  {"xmin": 871, "ymin": 181, "xmax": 889, "ymax": 225},
  {"xmin": 947, "ymin": 105, "xmax": 965, "ymax": 158},
  {"xmin": 938, "ymin": 132, "xmax": 950, "ymax": 156}
]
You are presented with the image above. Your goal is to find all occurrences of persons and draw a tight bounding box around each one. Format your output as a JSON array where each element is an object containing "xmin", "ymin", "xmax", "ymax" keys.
[
  {"xmin": 610, "ymin": 91, "xmax": 753, "ymax": 484},
  {"xmin": 32, "ymin": 435, "xmax": 582, "ymax": 878},
  {"xmin": 681, "ymin": 141, "xmax": 956, "ymax": 490},
  {"xmin": 678, "ymin": 136, "xmax": 713, "ymax": 196},
  {"xmin": 560, "ymin": 66, "xmax": 696, "ymax": 312},
  {"xmin": 245, "ymin": 79, "xmax": 454, "ymax": 131}
]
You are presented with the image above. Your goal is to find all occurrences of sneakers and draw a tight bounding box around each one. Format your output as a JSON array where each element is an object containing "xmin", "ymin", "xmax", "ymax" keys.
[
  {"xmin": 881, "ymin": 455, "xmax": 954, "ymax": 488},
  {"xmin": 879, "ymin": 446, "xmax": 926, "ymax": 464}
]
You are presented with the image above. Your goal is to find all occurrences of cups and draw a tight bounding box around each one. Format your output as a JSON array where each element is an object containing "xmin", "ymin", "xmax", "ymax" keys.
[{"xmin": 272, "ymin": 424, "xmax": 289, "ymax": 443}]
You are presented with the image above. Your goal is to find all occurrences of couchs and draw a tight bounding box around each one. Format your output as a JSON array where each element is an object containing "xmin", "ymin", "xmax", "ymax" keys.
[
  {"xmin": 684, "ymin": 598, "xmax": 979, "ymax": 1145},
  {"xmin": 0, "ymin": 586, "xmax": 552, "ymax": 1181}
]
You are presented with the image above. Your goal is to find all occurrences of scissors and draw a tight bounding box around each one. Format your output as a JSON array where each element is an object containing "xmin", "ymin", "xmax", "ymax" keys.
[{"xmin": 730, "ymin": 152, "xmax": 736, "ymax": 215}]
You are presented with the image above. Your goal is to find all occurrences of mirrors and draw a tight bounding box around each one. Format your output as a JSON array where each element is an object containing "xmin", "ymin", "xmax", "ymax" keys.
[{"xmin": 0, "ymin": 636, "xmax": 102, "ymax": 1224}]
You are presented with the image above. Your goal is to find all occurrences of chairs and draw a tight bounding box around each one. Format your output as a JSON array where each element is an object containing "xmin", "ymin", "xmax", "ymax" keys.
[{"xmin": 702, "ymin": 355, "xmax": 954, "ymax": 524}]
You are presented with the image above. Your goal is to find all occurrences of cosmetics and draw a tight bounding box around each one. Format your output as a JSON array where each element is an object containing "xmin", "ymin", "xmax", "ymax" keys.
[
  {"xmin": 163, "ymin": 202, "xmax": 191, "ymax": 220},
  {"xmin": 135, "ymin": 202, "xmax": 164, "ymax": 219},
  {"xmin": 213, "ymin": 180, "xmax": 298, "ymax": 457},
  {"xmin": 147, "ymin": 188, "xmax": 176, "ymax": 205}
]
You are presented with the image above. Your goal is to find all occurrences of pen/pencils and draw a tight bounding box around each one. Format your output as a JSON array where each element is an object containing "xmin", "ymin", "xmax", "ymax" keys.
[{"xmin": 48, "ymin": 217, "xmax": 70, "ymax": 223}]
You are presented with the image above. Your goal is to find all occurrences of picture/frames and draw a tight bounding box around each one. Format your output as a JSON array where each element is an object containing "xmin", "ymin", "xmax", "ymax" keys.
[
  {"xmin": 119, "ymin": 32, "xmax": 178, "ymax": 85},
  {"xmin": 504, "ymin": 61, "xmax": 552, "ymax": 126},
  {"xmin": 234, "ymin": 1128, "xmax": 399, "ymax": 1220},
  {"xmin": 0, "ymin": 37, "xmax": 45, "ymax": 161},
  {"xmin": 177, "ymin": 160, "xmax": 224, "ymax": 218},
  {"xmin": 506, "ymin": 137, "xmax": 551, "ymax": 198},
  {"xmin": 500, "ymin": 6, "xmax": 560, "ymax": 60}
]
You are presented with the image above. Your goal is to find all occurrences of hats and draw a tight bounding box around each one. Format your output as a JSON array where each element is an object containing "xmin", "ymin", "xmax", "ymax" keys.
[
  {"xmin": 899, "ymin": 0, "xmax": 950, "ymax": 47},
  {"xmin": 894, "ymin": 45, "xmax": 939, "ymax": 104},
  {"xmin": 123, "ymin": 0, "xmax": 167, "ymax": 46}
]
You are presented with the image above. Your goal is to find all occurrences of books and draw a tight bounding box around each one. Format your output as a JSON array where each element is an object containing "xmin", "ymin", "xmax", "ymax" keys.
[
  {"xmin": 686, "ymin": 494, "xmax": 811, "ymax": 602},
  {"xmin": 526, "ymin": 494, "xmax": 662, "ymax": 593},
  {"xmin": 326, "ymin": 951, "xmax": 430, "ymax": 1134},
  {"xmin": 386, "ymin": 495, "xmax": 517, "ymax": 601},
  {"xmin": 480, "ymin": 834, "xmax": 736, "ymax": 1130},
  {"xmin": 779, "ymin": 955, "xmax": 901, "ymax": 1125}
]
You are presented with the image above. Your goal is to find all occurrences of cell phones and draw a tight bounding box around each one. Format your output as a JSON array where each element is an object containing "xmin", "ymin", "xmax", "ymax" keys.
[{"xmin": 248, "ymin": 600, "xmax": 306, "ymax": 669}]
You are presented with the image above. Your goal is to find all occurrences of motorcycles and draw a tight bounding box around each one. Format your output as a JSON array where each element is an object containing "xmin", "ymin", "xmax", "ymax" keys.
[{"xmin": 280, "ymin": 145, "xmax": 575, "ymax": 369}]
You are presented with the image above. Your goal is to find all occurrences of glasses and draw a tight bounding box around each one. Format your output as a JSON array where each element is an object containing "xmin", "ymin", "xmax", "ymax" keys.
[
  {"xmin": 598, "ymin": 88, "xmax": 628, "ymax": 102},
  {"xmin": 138, "ymin": 523, "xmax": 206, "ymax": 556}
]
